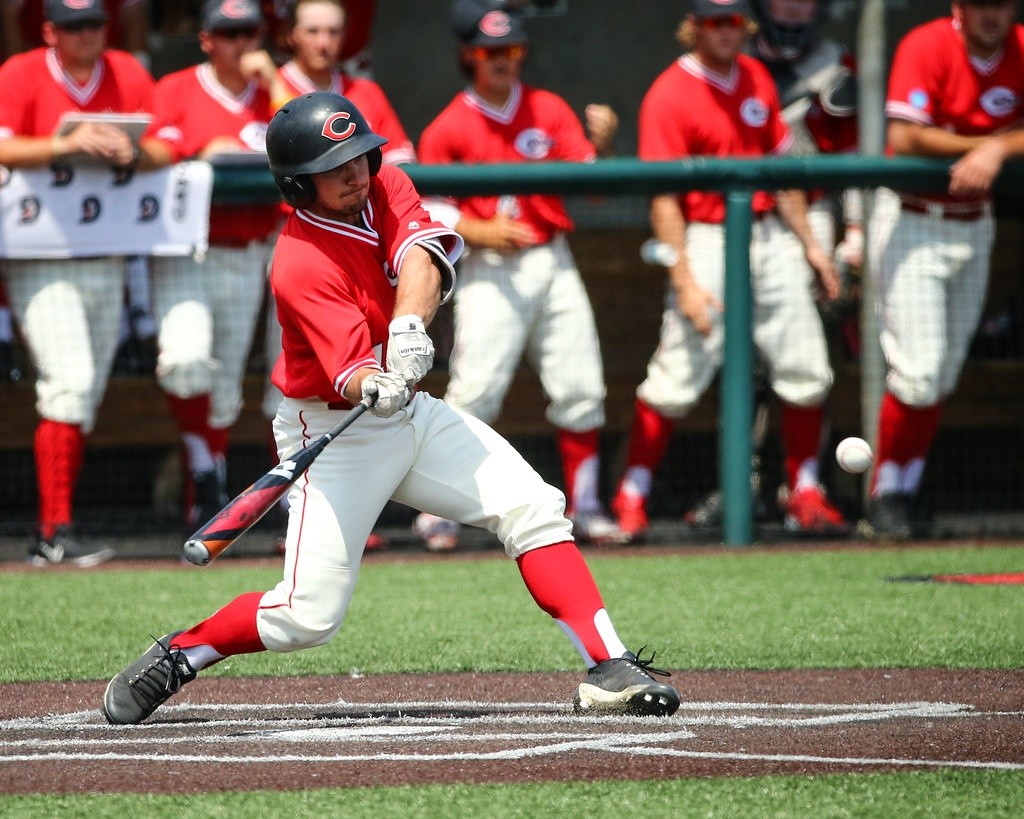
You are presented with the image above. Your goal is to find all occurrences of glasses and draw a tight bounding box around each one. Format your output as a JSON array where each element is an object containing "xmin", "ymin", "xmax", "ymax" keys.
[
  {"xmin": 61, "ymin": 19, "xmax": 104, "ymax": 31},
  {"xmin": 699, "ymin": 14, "xmax": 742, "ymax": 27},
  {"xmin": 212, "ymin": 24, "xmax": 257, "ymax": 40},
  {"xmin": 471, "ymin": 46, "xmax": 523, "ymax": 62}
]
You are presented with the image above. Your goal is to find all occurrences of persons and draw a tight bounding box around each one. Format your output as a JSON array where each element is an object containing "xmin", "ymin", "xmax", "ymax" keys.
[
  {"xmin": 611, "ymin": 0, "xmax": 865, "ymax": 537},
  {"xmin": 0, "ymin": 0, "xmax": 415, "ymax": 567},
  {"xmin": 102, "ymin": 91, "xmax": 678, "ymax": 724},
  {"xmin": 866, "ymin": 0, "xmax": 1024, "ymax": 543},
  {"xmin": 418, "ymin": 11, "xmax": 628, "ymax": 550}
]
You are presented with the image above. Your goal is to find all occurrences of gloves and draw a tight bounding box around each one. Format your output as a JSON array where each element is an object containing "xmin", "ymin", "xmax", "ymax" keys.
[
  {"xmin": 386, "ymin": 314, "xmax": 435, "ymax": 387},
  {"xmin": 361, "ymin": 372, "xmax": 409, "ymax": 417}
]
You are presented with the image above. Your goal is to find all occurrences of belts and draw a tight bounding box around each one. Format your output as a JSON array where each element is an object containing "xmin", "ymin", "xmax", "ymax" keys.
[
  {"xmin": 329, "ymin": 401, "xmax": 354, "ymax": 410},
  {"xmin": 901, "ymin": 201, "xmax": 982, "ymax": 219}
]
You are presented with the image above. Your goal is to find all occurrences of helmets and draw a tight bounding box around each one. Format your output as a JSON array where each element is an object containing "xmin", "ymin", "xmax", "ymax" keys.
[{"xmin": 266, "ymin": 91, "xmax": 388, "ymax": 208}]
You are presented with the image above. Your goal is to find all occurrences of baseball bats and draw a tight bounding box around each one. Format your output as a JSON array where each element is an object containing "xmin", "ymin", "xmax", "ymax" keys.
[{"xmin": 183, "ymin": 391, "xmax": 381, "ymax": 566}]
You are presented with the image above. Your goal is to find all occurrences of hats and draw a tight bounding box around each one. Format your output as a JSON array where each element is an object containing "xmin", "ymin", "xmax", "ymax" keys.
[
  {"xmin": 693, "ymin": 0, "xmax": 747, "ymax": 18},
  {"xmin": 202, "ymin": 0, "xmax": 261, "ymax": 32},
  {"xmin": 46, "ymin": 0, "xmax": 104, "ymax": 24},
  {"xmin": 455, "ymin": 6, "xmax": 527, "ymax": 47}
]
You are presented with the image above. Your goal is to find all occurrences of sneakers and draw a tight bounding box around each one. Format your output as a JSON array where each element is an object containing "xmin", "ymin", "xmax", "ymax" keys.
[
  {"xmin": 577, "ymin": 645, "xmax": 680, "ymax": 715},
  {"xmin": 103, "ymin": 630, "xmax": 197, "ymax": 724}
]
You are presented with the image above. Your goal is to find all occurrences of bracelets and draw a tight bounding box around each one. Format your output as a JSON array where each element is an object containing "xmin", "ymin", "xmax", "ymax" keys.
[{"xmin": 132, "ymin": 140, "xmax": 139, "ymax": 164}]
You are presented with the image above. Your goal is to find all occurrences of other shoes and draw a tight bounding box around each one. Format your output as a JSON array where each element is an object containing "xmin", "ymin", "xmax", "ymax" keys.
[
  {"xmin": 410, "ymin": 511, "xmax": 458, "ymax": 551},
  {"xmin": 567, "ymin": 509, "xmax": 633, "ymax": 543},
  {"xmin": 864, "ymin": 490, "xmax": 919, "ymax": 541},
  {"xmin": 776, "ymin": 481, "xmax": 843, "ymax": 535},
  {"xmin": 30, "ymin": 524, "xmax": 114, "ymax": 567},
  {"xmin": 614, "ymin": 492, "xmax": 660, "ymax": 544}
]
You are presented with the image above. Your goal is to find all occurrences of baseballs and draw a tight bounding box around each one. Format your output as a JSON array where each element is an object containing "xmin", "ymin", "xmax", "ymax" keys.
[{"xmin": 836, "ymin": 436, "xmax": 873, "ymax": 473}]
